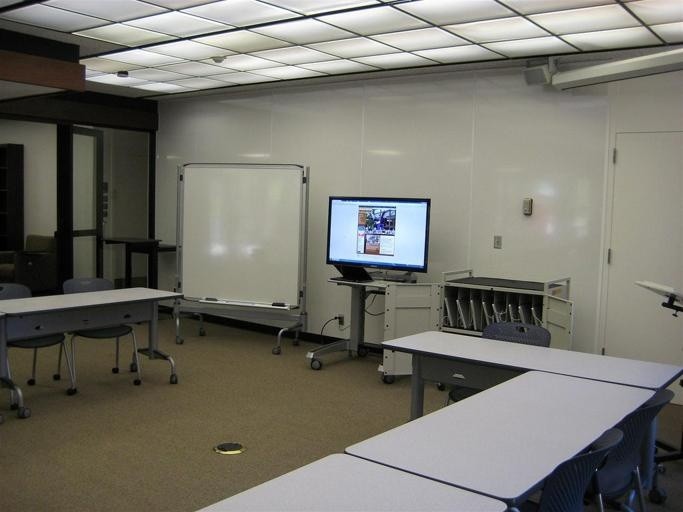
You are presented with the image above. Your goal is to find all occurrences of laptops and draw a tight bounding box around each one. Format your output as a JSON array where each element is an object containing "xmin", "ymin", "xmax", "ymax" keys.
[{"xmin": 331, "ymin": 264, "xmax": 374, "ymax": 283}]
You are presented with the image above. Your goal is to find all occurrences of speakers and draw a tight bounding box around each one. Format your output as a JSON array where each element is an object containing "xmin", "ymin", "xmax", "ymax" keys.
[{"xmin": 523, "ymin": 64, "xmax": 552, "ymax": 86}]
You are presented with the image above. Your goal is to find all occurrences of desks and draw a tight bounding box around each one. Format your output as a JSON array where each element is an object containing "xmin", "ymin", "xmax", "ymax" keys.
[
  {"xmin": 343, "ymin": 331, "xmax": 683, "ymax": 510},
  {"xmin": 103, "ymin": 235, "xmax": 178, "ymax": 289},
  {"xmin": 0, "ymin": 286, "xmax": 185, "ymax": 422},
  {"xmin": 307, "ymin": 277, "xmax": 416, "ymax": 370},
  {"xmin": 197, "ymin": 453, "xmax": 508, "ymax": 510}
]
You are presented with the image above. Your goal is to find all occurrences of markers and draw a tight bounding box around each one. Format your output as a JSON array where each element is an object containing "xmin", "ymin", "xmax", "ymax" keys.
[{"xmin": 205, "ymin": 298, "xmax": 217, "ymax": 301}]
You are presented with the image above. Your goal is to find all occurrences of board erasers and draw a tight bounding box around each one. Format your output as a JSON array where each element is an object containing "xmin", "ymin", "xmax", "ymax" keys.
[{"xmin": 272, "ymin": 303, "xmax": 285, "ymax": 306}]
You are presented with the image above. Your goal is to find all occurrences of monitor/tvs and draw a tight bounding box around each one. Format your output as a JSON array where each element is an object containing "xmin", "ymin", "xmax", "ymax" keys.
[{"xmin": 326, "ymin": 196, "xmax": 431, "ymax": 280}]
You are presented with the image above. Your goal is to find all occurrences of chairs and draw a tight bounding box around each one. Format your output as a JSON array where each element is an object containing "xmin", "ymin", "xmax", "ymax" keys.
[
  {"xmin": 1, "ymin": 277, "xmax": 143, "ymax": 396},
  {"xmin": 1, "ymin": 234, "xmax": 57, "ymax": 291},
  {"xmin": 446, "ymin": 321, "xmax": 551, "ymax": 405}
]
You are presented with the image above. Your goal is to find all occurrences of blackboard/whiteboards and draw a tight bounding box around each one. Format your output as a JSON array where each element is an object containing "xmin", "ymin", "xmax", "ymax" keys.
[{"xmin": 176, "ymin": 163, "xmax": 306, "ymax": 311}]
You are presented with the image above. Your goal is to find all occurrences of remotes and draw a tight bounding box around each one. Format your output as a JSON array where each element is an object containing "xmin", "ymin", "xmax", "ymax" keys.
[
  {"xmin": 411, "ymin": 277, "xmax": 417, "ymax": 283},
  {"xmin": 385, "ymin": 277, "xmax": 406, "ymax": 282}
]
